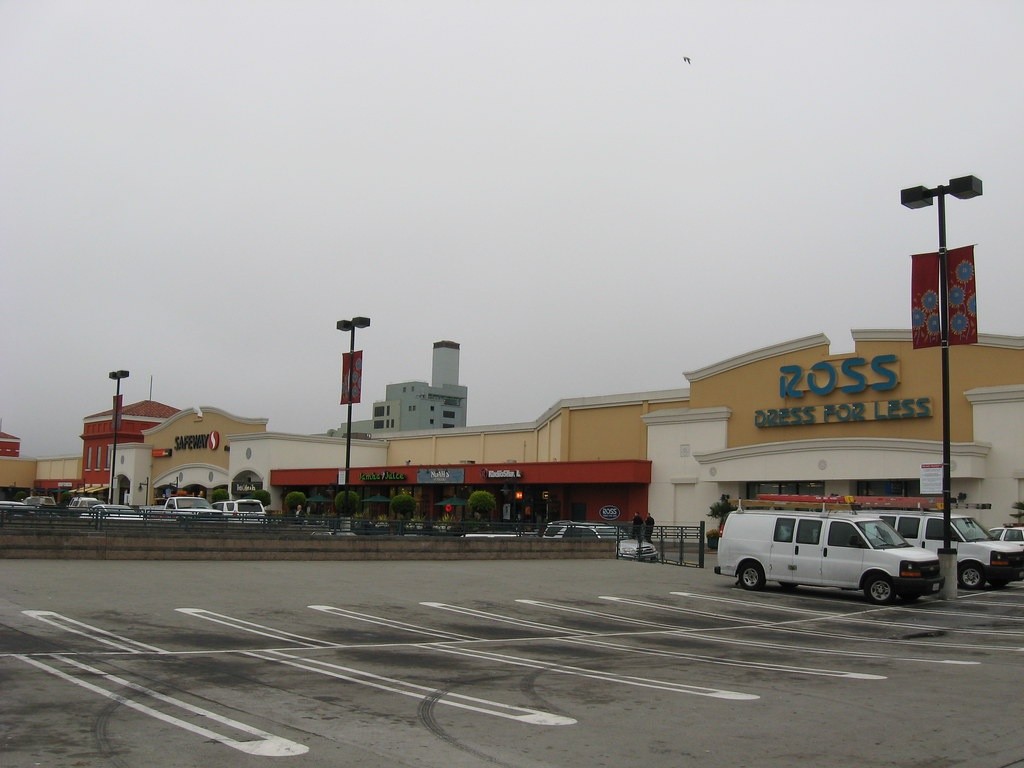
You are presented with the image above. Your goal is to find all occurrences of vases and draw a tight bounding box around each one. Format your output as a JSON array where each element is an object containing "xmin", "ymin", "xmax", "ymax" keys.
[{"xmin": 707, "ymin": 536, "xmax": 719, "ymax": 548}]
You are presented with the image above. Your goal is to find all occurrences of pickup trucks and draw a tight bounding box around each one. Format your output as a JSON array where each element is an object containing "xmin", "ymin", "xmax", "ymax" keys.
[{"xmin": 143, "ymin": 497, "xmax": 223, "ymax": 521}]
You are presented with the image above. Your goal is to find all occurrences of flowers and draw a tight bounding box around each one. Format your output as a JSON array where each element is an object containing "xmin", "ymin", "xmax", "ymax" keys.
[{"xmin": 706, "ymin": 529, "xmax": 720, "ymax": 537}]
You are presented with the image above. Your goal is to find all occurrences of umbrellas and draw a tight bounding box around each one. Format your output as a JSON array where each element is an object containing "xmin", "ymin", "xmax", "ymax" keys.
[
  {"xmin": 306, "ymin": 495, "xmax": 335, "ymax": 515},
  {"xmin": 434, "ymin": 495, "xmax": 466, "ymax": 513},
  {"xmin": 361, "ymin": 494, "xmax": 392, "ymax": 515}
]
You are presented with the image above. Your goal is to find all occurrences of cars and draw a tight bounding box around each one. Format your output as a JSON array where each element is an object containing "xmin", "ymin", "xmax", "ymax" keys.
[
  {"xmin": 69, "ymin": 497, "xmax": 104, "ymax": 509},
  {"xmin": 81, "ymin": 504, "xmax": 142, "ymax": 520},
  {"xmin": 989, "ymin": 524, "xmax": 1024, "ymax": 546}
]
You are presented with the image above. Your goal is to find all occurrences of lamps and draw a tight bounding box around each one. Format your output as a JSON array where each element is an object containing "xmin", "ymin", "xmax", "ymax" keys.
[{"xmin": 951, "ymin": 492, "xmax": 967, "ymax": 504}]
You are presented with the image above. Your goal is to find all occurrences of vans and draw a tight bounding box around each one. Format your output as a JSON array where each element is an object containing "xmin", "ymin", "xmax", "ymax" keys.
[
  {"xmin": 824, "ymin": 509, "xmax": 1024, "ymax": 590},
  {"xmin": 21, "ymin": 496, "xmax": 57, "ymax": 506},
  {"xmin": 715, "ymin": 507, "xmax": 943, "ymax": 604}
]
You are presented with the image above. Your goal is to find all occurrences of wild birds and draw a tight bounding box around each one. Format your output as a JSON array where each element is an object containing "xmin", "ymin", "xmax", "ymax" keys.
[{"xmin": 683, "ymin": 56, "xmax": 692, "ymax": 64}]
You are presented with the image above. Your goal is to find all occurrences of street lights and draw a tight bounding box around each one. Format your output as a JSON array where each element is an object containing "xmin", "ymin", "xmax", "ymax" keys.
[
  {"xmin": 902, "ymin": 174, "xmax": 982, "ymax": 600},
  {"xmin": 108, "ymin": 370, "xmax": 129, "ymax": 503},
  {"xmin": 336, "ymin": 316, "xmax": 371, "ymax": 508},
  {"xmin": 169, "ymin": 484, "xmax": 179, "ymax": 496},
  {"xmin": 138, "ymin": 483, "xmax": 148, "ymax": 506}
]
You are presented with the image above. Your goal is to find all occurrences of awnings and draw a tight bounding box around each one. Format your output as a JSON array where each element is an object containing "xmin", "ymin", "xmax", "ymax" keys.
[{"xmin": 68, "ymin": 486, "xmax": 108, "ymax": 493}]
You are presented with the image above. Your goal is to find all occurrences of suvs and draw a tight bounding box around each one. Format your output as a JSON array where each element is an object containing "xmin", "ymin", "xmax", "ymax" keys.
[
  {"xmin": 543, "ymin": 521, "xmax": 658, "ymax": 561},
  {"xmin": 212, "ymin": 500, "xmax": 267, "ymax": 523}
]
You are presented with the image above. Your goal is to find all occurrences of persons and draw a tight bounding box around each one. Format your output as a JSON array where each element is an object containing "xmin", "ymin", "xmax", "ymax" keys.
[
  {"xmin": 295, "ymin": 505, "xmax": 306, "ymax": 525},
  {"xmin": 632, "ymin": 512, "xmax": 643, "ymax": 540},
  {"xmin": 644, "ymin": 513, "xmax": 654, "ymax": 544}
]
